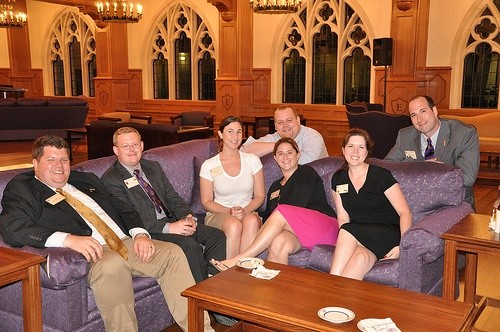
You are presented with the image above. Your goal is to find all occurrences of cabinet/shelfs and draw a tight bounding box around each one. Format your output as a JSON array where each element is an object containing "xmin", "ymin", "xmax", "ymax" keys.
[{"xmin": 374, "ymin": 66, "xmax": 452, "ymax": 109}]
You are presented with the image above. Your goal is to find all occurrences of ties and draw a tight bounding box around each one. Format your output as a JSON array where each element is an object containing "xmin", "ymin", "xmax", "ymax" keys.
[
  {"xmin": 425, "ymin": 138, "xmax": 434, "ymax": 160},
  {"xmin": 133, "ymin": 169, "xmax": 175, "ymax": 223},
  {"xmin": 56, "ymin": 188, "xmax": 129, "ymax": 262}
]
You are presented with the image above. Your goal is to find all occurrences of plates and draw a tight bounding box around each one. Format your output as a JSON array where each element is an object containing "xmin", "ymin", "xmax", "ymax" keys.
[
  {"xmin": 317, "ymin": 307, "xmax": 356, "ymax": 324},
  {"xmin": 357, "ymin": 318, "xmax": 381, "ymax": 332},
  {"xmin": 235, "ymin": 257, "xmax": 265, "ymax": 269}
]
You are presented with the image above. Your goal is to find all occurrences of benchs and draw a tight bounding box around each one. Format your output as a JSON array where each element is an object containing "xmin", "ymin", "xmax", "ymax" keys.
[{"xmin": 255, "ymin": 114, "xmax": 305, "ymax": 137}]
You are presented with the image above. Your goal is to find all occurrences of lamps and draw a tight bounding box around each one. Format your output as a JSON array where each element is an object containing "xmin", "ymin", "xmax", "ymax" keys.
[
  {"xmin": 95, "ymin": 2, "xmax": 143, "ymax": 23},
  {"xmin": 0, "ymin": 11, "xmax": 27, "ymax": 29},
  {"xmin": 248, "ymin": 0, "xmax": 299, "ymax": 15}
]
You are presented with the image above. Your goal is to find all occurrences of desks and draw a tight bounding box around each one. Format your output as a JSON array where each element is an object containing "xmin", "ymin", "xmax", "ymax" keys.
[
  {"xmin": 66, "ymin": 130, "xmax": 86, "ymax": 161},
  {"xmin": 0, "ymin": 89, "xmax": 25, "ymax": 98}
]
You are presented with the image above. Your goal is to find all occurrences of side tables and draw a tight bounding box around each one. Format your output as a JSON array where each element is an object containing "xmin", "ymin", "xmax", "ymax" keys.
[
  {"xmin": 439, "ymin": 213, "xmax": 500, "ymax": 304},
  {"xmin": 0, "ymin": 247, "xmax": 47, "ymax": 332}
]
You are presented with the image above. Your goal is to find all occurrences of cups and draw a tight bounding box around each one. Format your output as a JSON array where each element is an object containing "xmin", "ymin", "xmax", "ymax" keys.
[{"xmin": 182, "ymin": 217, "xmax": 196, "ymax": 227}]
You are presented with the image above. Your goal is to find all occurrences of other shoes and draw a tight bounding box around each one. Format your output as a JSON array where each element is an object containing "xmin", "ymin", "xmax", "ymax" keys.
[
  {"xmin": 209, "ymin": 259, "xmax": 230, "ymax": 273},
  {"xmin": 211, "ymin": 311, "xmax": 239, "ymax": 326}
]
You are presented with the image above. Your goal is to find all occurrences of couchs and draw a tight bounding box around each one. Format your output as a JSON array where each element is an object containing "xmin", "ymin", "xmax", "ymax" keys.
[
  {"xmin": 169, "ymin": 111, "xmax": 216, "ymax": 125},
  {"xmin": 345, "ymin": 101, "xmax": 412, "ymax": 160},
  {"xmin": 0, "ymin": 139, "xmax": 475, "ymax": 332},
  {"xmin": 97, "ymin": 112, "xmax": 151, "ymax": 123},
  {"xmin": 86, "ymin": 120, "xmax": 210, "ymax": 161},
  {"xmin": 438, "ymin": 113, "xmax": 500, "ymax": 169},
  {"xmin": 0, "ymin": 98, "xmax": 89, "ymax": 130}
]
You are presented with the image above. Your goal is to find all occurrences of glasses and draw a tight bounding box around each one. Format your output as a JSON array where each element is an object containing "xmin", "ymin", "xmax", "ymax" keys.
[{"xmin": 113, "ymin": 142, "xmax": 141, "ymax": 151}]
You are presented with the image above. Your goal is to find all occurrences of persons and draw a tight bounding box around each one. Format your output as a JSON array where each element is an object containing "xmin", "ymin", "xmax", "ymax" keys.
[
  {"xmin": 329, "ymin": 129, "xmax": 412, "ymax": 281},
  {"xmin": 384, "ymin": 95, "xmax": 480, "ymax": 210},
  {"xmin": 198, "ymin": 116, "xmax": 265, "ymax": 259},
  {"xmin": 0, "ymin": 135, "xmax": 216, "ymax": 332},
  {"xmin": 209, "ymin": 137, "xmax": 339, "ymax": 265},
  {"xmin": 100, "ymin": 126, "xmax": 227, "ymax": 284},
  {"xmin": 240, "ymin": 106, "xmax": 329, "ymax": 165}
]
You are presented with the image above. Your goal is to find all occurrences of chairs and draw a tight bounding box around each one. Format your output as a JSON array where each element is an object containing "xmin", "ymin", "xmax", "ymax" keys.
[{"xmin": 243, "ymin": 121, "xmax": 256, "ymax": 138}]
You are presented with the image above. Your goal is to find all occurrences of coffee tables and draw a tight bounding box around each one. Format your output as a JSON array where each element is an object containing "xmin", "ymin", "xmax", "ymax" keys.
[{"xmin": 180, "ymin": 260, "xmax": 479, "ymax": 332}]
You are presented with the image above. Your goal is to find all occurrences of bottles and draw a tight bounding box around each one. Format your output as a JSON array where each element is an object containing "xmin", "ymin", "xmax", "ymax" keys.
[{"xmin": 492, "ymin": 185, "xmax": 500, "ymax": 233}]
